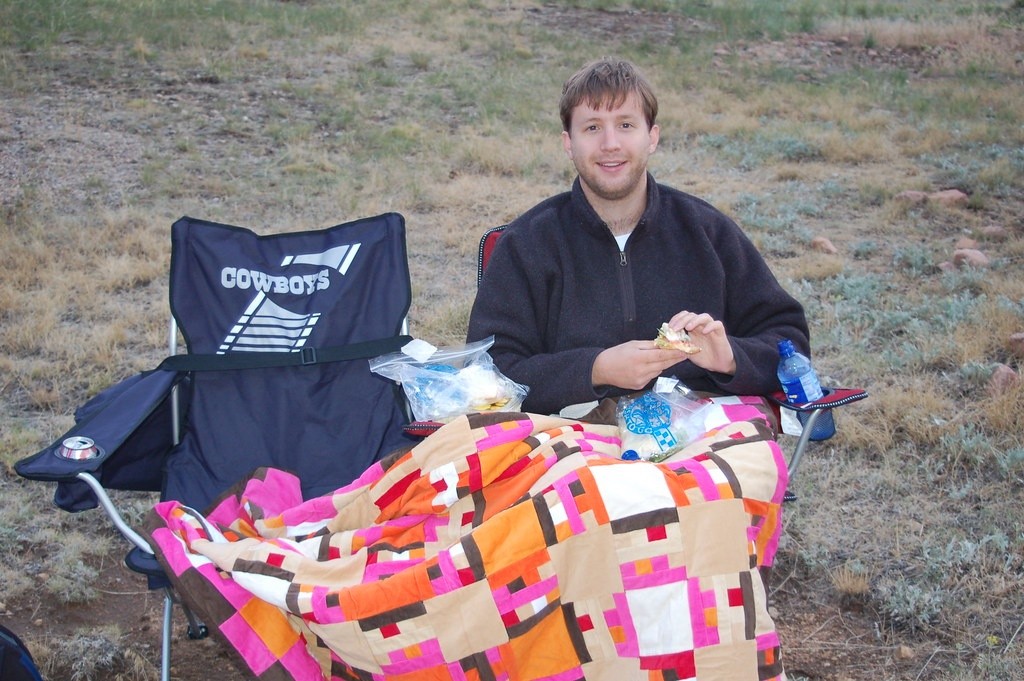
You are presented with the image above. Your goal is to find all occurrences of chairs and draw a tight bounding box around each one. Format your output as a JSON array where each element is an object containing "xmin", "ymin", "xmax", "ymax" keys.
[
  {"xmin": 399, "ymin": 222, "xmax": 873, "ymax": 505},
  {"xmin": 12, "ymin": 210, "xmax": 429, "ymax": 681}
]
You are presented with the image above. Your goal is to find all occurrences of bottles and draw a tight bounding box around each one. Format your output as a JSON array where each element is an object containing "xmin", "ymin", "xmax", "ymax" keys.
[{"xmin": 775, "ymin": 339, "xmax": 836, "ymax": 441}]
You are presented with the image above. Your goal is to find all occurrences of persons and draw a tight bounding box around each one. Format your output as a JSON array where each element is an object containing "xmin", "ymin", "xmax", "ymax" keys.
[{"xmin": 467, "ymin": 57, "xmax": 811, "ymax": 417}]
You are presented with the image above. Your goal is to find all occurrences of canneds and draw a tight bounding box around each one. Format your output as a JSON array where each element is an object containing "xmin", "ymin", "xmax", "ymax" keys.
[{"xmin": 62, "ymin": 437, "xmax": 97, "ymax": 462}]
